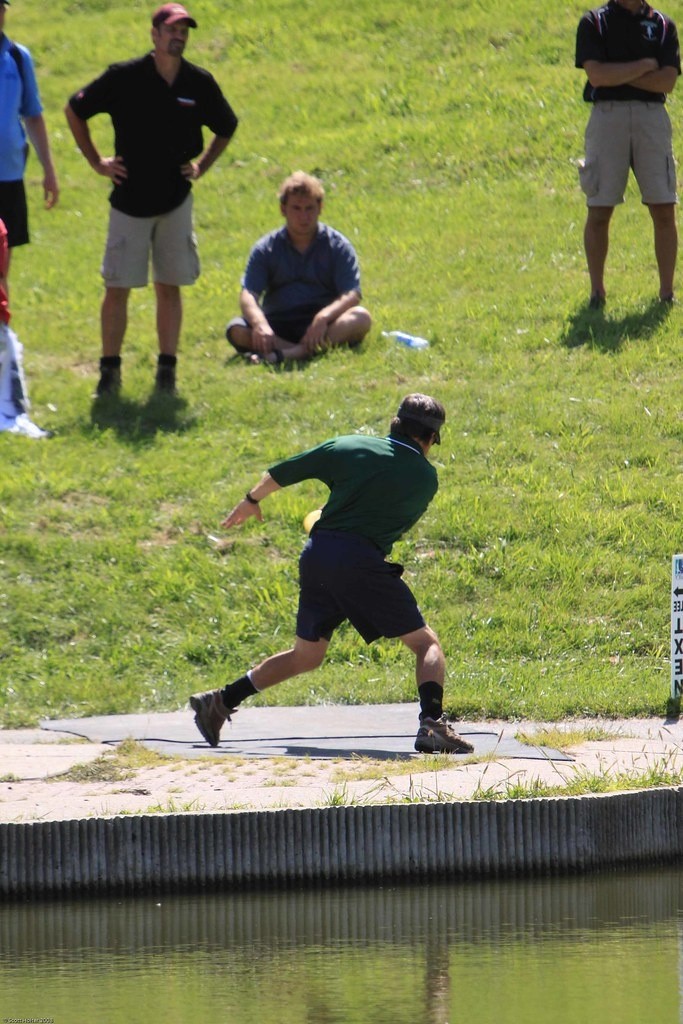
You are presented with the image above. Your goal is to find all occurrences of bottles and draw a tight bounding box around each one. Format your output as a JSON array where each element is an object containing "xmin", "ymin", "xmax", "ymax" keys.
[{"xmin": 381, "ymin": 329, "xmax": 429, "ymax": 352}]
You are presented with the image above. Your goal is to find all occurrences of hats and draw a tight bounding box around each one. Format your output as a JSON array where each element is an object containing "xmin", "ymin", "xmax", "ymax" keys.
[
  {"xmin": 152, "ymin": 3, "xmax": 195, "ymax": 28},
  {"xmin": 0, "ymin": 0, "xmax": 11, "ymax": 7},
  {"xmin": 398, "ymin": 404, "xmax": 443, "ymax": 445}
]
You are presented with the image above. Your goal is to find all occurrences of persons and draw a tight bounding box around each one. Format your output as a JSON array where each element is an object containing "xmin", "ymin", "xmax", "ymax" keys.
[
  {"xmin": 0, "ymin": 0, "xmax": 60, "ymax": 441},
  {"xmin": 189, "ymin": 394, "xmax": 474, "ymax": 755},
  {"xmin": 226, "ymin": 171, "xmax": 372, "ymax": 365},
  {"xmin": 574, "ymin": 0, "xmax": 680, "ymax": 315},
  {"xmin": 65, "ymin": 2, "xmax": 239, "ymax": 404}
]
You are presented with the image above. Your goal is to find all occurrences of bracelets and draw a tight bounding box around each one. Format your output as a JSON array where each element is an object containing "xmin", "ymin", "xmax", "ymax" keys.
[{"xmin": 246, "ymin": 493, "xmax": 259, "ymax": 504}]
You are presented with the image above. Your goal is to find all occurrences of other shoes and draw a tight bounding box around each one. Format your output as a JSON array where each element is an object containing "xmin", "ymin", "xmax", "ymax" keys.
[
  {"xmin": 244, "ymin": 349, "xmax": 283, "ymax": 365},
  {"xmin": 0, "ymin": 415, "xmax": 53, "ymax": 439},
  {"xmin": 662, "ymin": 294, "xmax": 678, "ymax": 305},
  {"xmin": 95, "ymin": 366, "xmax": 121, "ymax": 399},
  {"xmin": 588, "ymin": 289, "xmax": 606, "ymax": 311},
  {"xmin": 152, "ymin": 370, "xmax": 177, "ymax": 396}
]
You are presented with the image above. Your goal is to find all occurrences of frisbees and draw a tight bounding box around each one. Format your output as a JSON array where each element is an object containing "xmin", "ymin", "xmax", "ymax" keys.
[{"xmin": 302, "ymin": 508, "xmax": 322, "ymax": 535}]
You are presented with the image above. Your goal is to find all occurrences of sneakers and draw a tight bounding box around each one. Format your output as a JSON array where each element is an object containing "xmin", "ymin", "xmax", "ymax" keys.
[
  {"xmin": 190, "ymin": 689, "xmax": 238, "ymax": 746},
  {"xmin": 415, "ymin": 712, "xmax": 475, "ymax": 753}
]
